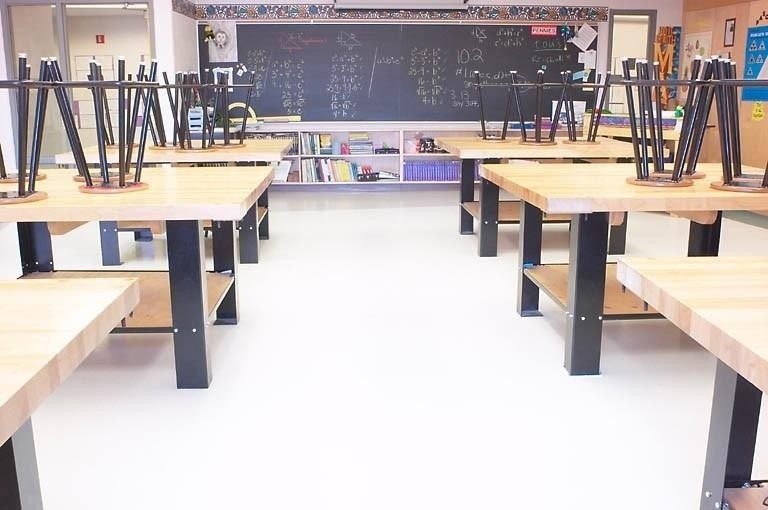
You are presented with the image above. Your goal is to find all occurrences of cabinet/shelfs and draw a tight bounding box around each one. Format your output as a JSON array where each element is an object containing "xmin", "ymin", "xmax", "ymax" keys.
[{"xmin": 240, "ymin": 126, "xmax": 483, "ymax": 193}]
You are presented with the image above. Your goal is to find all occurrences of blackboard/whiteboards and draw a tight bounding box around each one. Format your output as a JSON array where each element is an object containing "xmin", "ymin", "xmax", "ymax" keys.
[{"xmin": 196, "ymin": 19, "xmax": 598, "ymax": 124}]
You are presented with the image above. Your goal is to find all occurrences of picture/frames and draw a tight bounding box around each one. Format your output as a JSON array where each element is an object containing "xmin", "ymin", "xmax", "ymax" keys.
[
  {"xmin": 675, "ymin": 30, "xmax": 714, "ymax": 102},
  {"xmin": 724, "ymin": 18, "xmax": 736, "ymax": 47}
]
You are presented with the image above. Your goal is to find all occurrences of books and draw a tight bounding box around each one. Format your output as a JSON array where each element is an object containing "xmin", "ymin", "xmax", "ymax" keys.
[
  {"xmin": 296, "ymin": 131, "xmax": 400, "ymax": 182},
  {"xmin": 403, "ymin": 160, "xmax": 482, "ymax": 183}
]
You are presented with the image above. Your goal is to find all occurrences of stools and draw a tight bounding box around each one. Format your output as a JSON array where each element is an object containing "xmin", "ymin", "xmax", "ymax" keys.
[
  {"xmin": 474, "ymin": 71, "xmax": 519, "ymax": 143},
  {"xmin": 510, "ymin": 70, "xmax": 571, "ymax": 146},
  {"xmin": 622, "ymin": 54, "xmax": 768, "ymax": 193},
  {"xmin": 561, "ymin": 72, "xmax": 611, "ymax": 145},
  {"xmin": 0, "ymin": 53, "xmax": 255, "ymax": 204}
]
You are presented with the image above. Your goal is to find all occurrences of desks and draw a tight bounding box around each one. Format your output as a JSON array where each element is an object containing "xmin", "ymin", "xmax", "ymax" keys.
[
  {"xmin": 479, "ymin": 160, "xmax": 764, "ymax": 375},
  {"xmin": 1, "ymin": 170, "xmax": 276, "ymax": 388},
  {"xmin": 615, "ymin": 253, "xmax": 766, "ymax": 510},
  {"xmin": 55, "ymin": 139, "xmax": 294, "ymax": 266},
  {"xmin": 583, "ymin": 113, "xmax": 684, "ymax": 163},
  {"xmin": 436, "ymin": 134, "xmax": 673, "ymax": 258},
  {"xmin": 1, "ymin": 274, "xmax": 139, "ymax": 510}
]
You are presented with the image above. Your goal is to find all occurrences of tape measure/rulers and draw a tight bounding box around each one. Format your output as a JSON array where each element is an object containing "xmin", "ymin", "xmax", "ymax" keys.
[{"xmin": 228, "ymin": 102, "xmax": 302, "ymax": 122}]
